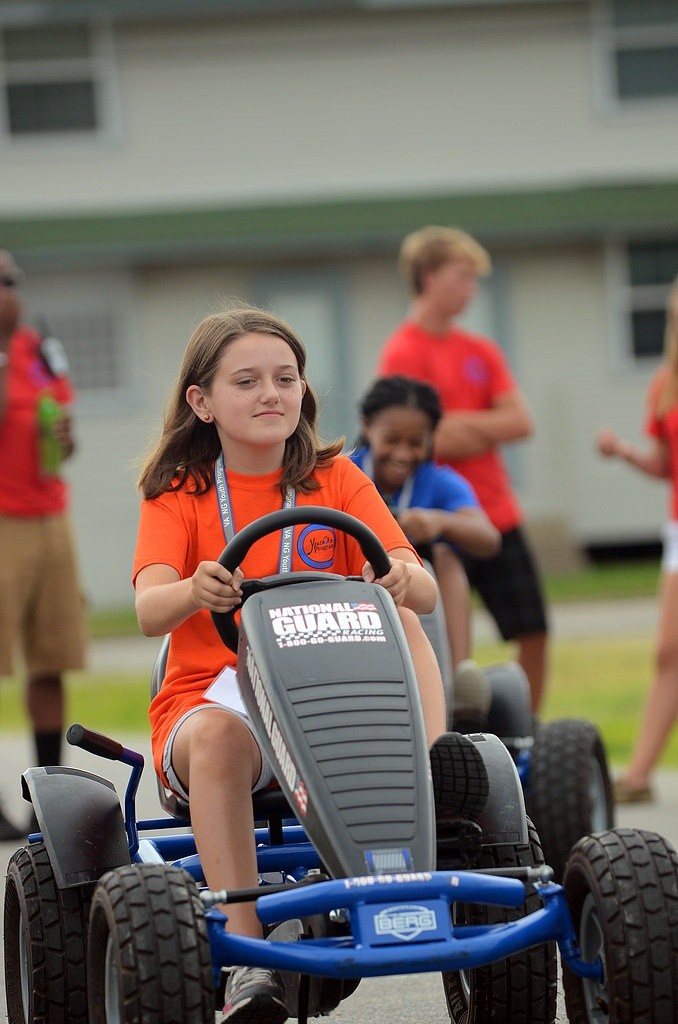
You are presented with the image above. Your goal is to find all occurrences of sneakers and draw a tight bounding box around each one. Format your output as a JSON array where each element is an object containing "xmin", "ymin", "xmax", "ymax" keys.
[
  {"xmin": 428, "ymin": 732, "xmax": 489, "ymax": 821},
  {"xmin": 218, "ymin": 965, "xmax": 289, "ymax": 1024}
]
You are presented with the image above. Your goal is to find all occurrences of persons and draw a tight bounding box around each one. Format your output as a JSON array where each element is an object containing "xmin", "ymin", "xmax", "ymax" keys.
[
  {"xmin": 344, "ymin": 374, "xmax": 500, "ymax": 672},
  {"xmin": 595, "ymin": 279, "xmax": 678, "ymax": 805},
  {"xmin": 379, "ymin": 226, "xmax": 553, "ymax": 737},
  {"xmin": 132, "ymin": 310, "xmax": 446, "ymax": 1024},
  {"xmin": 1, "ymin": 251, "xmax": 85, "ymax": 842}
]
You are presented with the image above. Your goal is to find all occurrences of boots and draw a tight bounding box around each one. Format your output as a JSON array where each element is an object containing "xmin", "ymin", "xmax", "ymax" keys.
[
  {"xmin": 23, "ymin": 729, "xmax": 61, "ymax": 838},
  {"xmin": 0, "ymin": 811, "xmax": 25, "ymax": 841}
]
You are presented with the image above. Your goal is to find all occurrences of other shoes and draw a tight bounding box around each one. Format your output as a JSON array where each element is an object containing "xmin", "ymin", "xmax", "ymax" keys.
[
  {"xmin": 452, "ymin": 657, "xmax": 492, "ymax": 721},
  {"xmin": 613, "ymin": 781, "xmax": 651, "ymax": 802}
]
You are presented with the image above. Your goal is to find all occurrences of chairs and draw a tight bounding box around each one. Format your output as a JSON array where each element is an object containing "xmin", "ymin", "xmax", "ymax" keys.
[{"xmin": 150, "ymin": 557, "xmax": 452, "ymax": 822}]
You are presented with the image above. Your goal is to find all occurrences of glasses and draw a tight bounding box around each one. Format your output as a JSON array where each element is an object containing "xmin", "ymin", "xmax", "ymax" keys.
[{"xmin": 0, "ymin": 270, "xmax": 21, "ymax": 286}]
